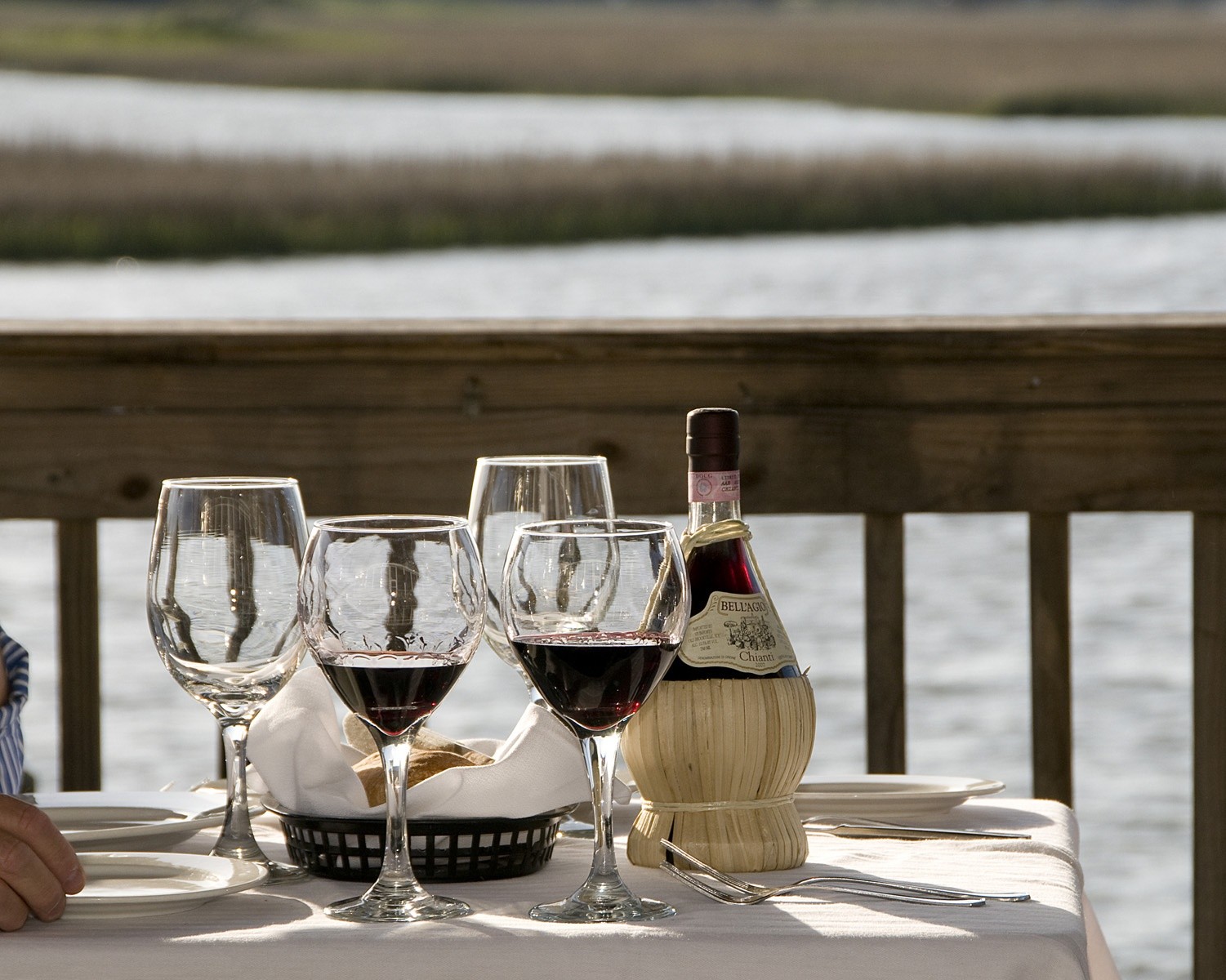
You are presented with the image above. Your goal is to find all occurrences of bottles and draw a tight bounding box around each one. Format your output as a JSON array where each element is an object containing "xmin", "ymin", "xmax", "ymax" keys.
[{"xmin": 626, "ymin": 404, "xmax": 806, "ymax": 687}]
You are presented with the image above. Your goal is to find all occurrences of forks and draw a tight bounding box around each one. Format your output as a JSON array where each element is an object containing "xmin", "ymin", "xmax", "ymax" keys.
[
  {"xmin": 654, "ymin": 861, "xmax": 987, "ymax": 914},
  {"xmin": 655, "ymin": 836, "xmax": 1032, "ymax": 906}
]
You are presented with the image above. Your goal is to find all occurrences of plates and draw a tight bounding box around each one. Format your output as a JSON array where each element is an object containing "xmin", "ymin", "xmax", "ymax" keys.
[
  {"xmin": 784, "ymin": 772, "xmax": 1005, "ymax": 815},
  {"xmin": 20, "ymin": 789, "xmax": 274, "ymax": 854},
  {"xmin": 38, "ymin": 845, "xmax": 273, "ymax": 920}
]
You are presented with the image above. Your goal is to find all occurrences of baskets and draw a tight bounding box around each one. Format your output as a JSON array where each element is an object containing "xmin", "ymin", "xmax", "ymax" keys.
[{"xmin": 259, "ymin": 790, "xmax": 582, "ymax": 884}]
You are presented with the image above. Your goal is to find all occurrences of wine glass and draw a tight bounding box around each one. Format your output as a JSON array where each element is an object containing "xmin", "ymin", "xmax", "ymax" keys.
[
  {"xmin": 501, "ymin": 507, "xmax": 676, "ymax": 925},
  {"xmin": 141, "ymin": 477, "xmax": 312, "ymax": 885},
  {"xmin": 296, "ymin": 514, "xmax": 492, "ymax": 924},
  {"xmin": 457, "ymin": 453, "xmax": 619, "ymax": 845}
]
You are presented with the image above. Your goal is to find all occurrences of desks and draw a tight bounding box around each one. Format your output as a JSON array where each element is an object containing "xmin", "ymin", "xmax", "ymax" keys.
[{"xmin": 0, "ymin": 790, "xmax": 1123, "ymax": 980}]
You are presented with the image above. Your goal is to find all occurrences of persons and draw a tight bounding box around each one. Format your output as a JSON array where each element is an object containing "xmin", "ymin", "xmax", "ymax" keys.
[{"xmin": 0, "ymin": 624, "xmax": 86, "ymax": 933}]
